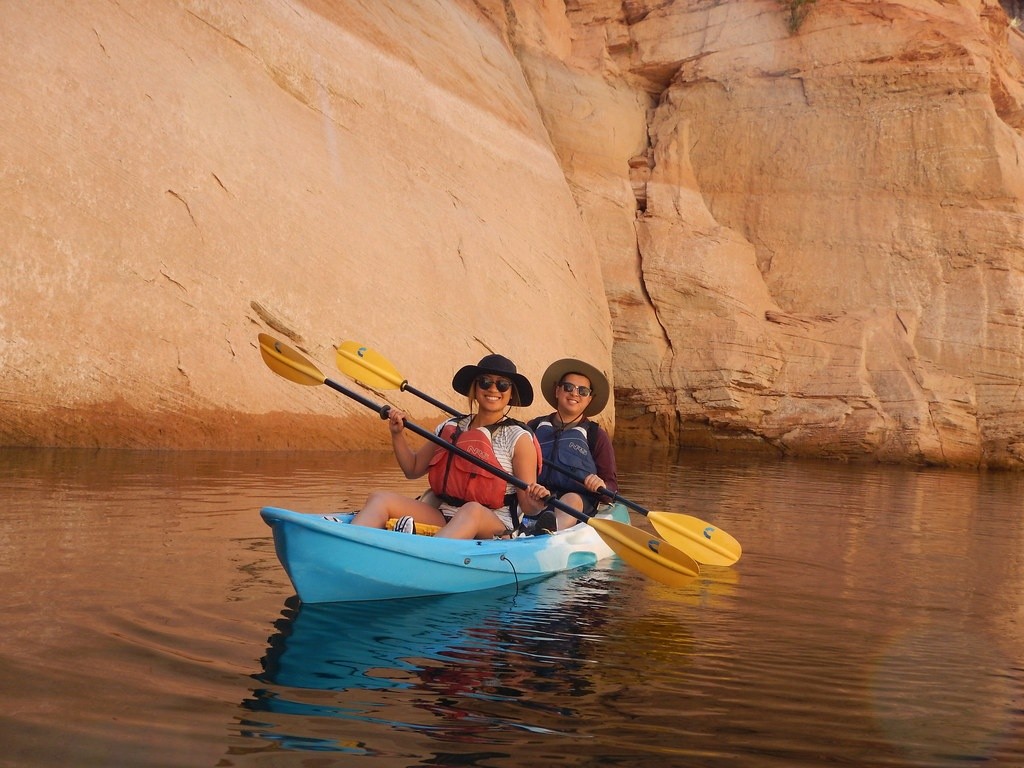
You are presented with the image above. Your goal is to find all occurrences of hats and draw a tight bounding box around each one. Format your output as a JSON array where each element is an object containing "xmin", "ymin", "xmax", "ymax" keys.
[
  {"xmin": 452, "ymin": 354, "xmax": 533, "ymax": 407},
  {"xmin": 540, "ymin": 358, "xmax": 609, "ymax": 417}
]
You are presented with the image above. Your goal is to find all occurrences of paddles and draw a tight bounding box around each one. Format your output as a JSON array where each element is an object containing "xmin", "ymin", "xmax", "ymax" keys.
[
  {"xmin": 256, "ymin": 332, "xmax": 701, "ymax": 588},
  {"xmin": 335, "ymin": 338, "xmax": 742, "ymax": 568}
]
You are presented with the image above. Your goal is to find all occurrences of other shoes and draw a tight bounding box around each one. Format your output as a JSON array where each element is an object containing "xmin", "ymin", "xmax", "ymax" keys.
[
  {"xmin": 535, "ymin": 511, "xmax": 557, "ymax": 535},
  {"xmin": 392, "ymin": 516, "xmax": 416, "ymax": 534}
]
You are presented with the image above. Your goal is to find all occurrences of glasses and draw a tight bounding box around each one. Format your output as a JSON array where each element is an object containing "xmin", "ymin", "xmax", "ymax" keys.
[
  {"xmin": 560, "ymin": 382, "xmax": 592, "ymax": 396},
  {"xmin": 475, "ymin": 376, "xmax": 513, "ymax": 392}
]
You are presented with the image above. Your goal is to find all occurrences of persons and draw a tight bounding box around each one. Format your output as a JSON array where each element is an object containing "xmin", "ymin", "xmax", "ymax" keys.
[
  {"xmin": 525, "ymin": 358, "xmax": 619, "ymax": 536},
  {"xmin": 348, "ymin": 354, "xmax": 551, "ymax": 540}
]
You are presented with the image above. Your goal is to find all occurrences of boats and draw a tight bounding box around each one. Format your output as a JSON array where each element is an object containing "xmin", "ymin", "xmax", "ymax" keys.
[
  {"xmin": 243, "ymin": 558, "xmax": 623, "ymax": 748},
  {"xmin": 257, "ymin": 501, "xmax": 635, "ymax": 605}
]
